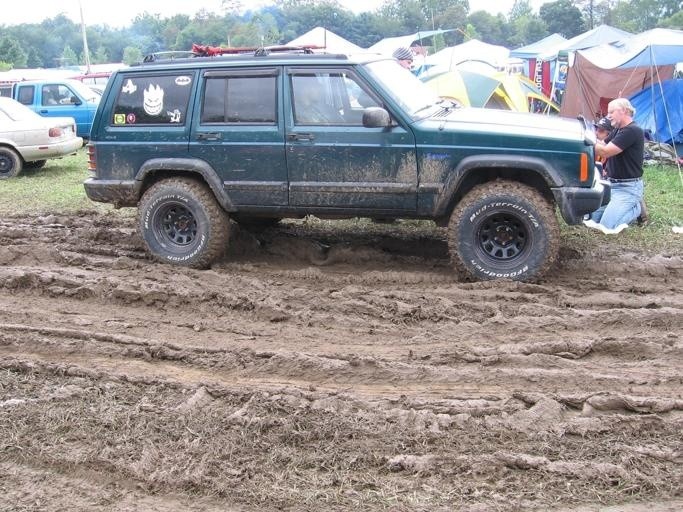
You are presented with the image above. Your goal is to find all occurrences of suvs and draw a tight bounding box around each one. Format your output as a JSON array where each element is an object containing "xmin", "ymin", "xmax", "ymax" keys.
[{"xmin": 85, "ymin": 47, "xmax": 610, "ymax": 281}]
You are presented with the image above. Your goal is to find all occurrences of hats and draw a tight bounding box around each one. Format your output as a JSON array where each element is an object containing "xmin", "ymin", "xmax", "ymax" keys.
[
  {"xmin": 594, "ymin": 118, "xmax": 613, "ymax": 130},
  {"xmin": 393, "ymin": 48, "xmax": 413, "ymax": 60}
]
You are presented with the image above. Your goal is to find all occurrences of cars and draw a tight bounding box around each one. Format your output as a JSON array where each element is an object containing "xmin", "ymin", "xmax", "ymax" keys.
[{"xmin": 0, "ymin": 92, "xmax": 83, "ymax": 178}]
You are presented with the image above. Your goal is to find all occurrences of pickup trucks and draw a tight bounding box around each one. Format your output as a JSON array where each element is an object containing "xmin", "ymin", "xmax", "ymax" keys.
[{"xmin": 2, "ymin": 72, "xmax": 115, "ymax": 137}]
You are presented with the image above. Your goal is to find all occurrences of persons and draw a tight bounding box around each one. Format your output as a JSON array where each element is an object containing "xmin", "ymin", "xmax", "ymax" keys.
[
  {"xmin": 45, "ymin": 85, "xmax": 69, "ymax": 105},
  {"xmin": 591, "ymin": 97, "xmax": 648, "ymax": 231},
  {"xmin": 357, "ymin": 47, "xmax": 411, "ymax": 224},
  {"xmin": 593, "ymin": 117, "xmax": 616, "ymax": 177}
]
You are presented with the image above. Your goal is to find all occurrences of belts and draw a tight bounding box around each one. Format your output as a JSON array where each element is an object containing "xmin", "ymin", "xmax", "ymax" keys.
[{"xmin": 611, "ymin": 179, "xmax": 638, "ymax": 182}]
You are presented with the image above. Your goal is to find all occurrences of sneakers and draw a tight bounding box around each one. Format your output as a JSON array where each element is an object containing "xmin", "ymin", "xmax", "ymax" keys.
[{"xmin": 637, "ymin": 201, "xmax": 647, "ymax": 227}]
